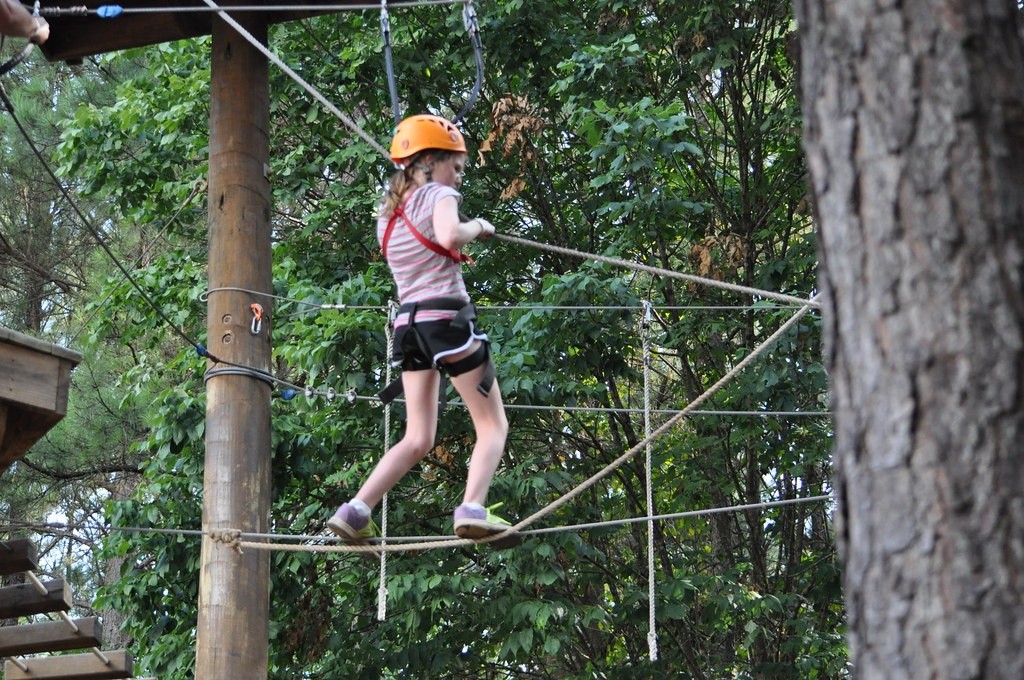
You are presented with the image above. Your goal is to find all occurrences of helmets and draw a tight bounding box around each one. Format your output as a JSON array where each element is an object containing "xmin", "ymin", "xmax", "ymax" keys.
[{"xmin": 390, "ymin": 114, "xmax": 467, "ymax": 164}]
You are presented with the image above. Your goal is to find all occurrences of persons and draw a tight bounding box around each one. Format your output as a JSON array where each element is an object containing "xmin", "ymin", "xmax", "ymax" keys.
[
  {"xmin": 325, "ymin": 115, "xmax": 522, "ymax": 549},
  {"xmin": 0, "ymin": 0, "xmax": 50, "ymax": 45}
]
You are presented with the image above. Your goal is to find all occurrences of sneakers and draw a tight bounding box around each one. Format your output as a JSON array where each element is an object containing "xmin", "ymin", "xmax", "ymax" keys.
[
  {"xmin": 328, "ymin": 502, "xmax": 382, "ymax": 561},
  {"xmin": 453, "ymin": 502, "xmax": 520, "ymax": 550}
]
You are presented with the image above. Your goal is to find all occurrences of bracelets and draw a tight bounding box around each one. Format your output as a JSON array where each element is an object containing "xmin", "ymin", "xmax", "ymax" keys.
[
  {"xmin": 32, "ymin": 17, "xmax": 40, "ymax": 36},
  {"xmin": 473, "ymin": 218, "xmax": 485, "ymax": 234}
]
